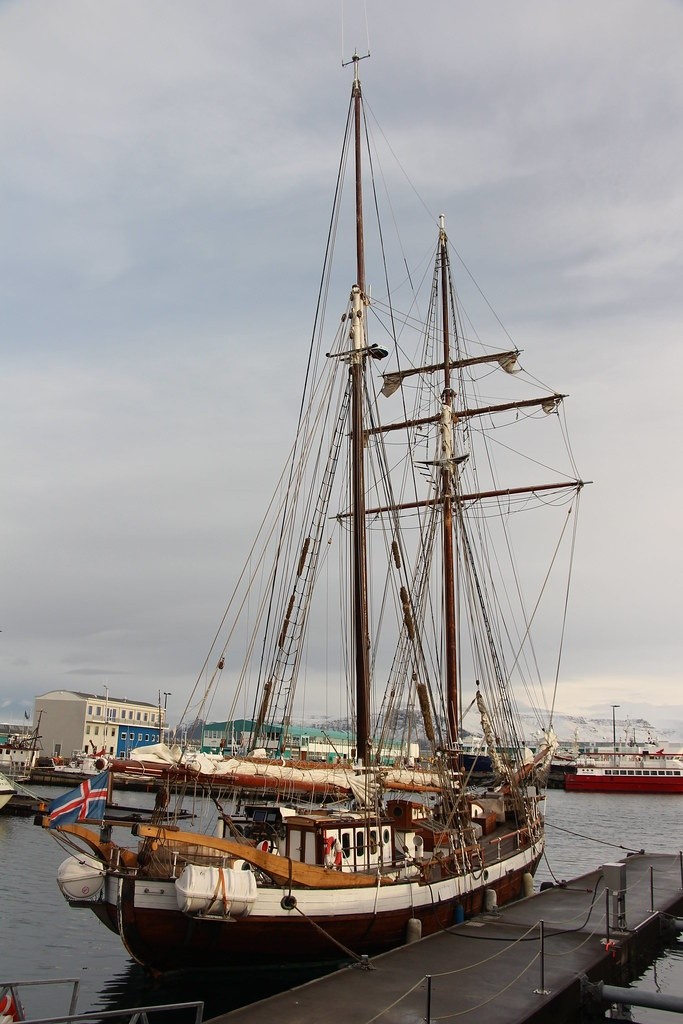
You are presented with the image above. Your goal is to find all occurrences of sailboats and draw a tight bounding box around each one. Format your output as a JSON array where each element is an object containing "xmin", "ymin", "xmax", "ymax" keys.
[{"xmin": 32, "ymin": 26, "xmax": 598, "ymax": 989}]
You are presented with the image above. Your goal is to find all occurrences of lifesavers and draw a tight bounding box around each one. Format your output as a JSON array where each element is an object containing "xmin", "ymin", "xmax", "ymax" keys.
[
  {"xmin": 605, "ymin": 942, "xmax": 616, "ymax": 959},
  {"xmin": 325, "ymin": 835, "xmax": 341, "ymax": 870}
]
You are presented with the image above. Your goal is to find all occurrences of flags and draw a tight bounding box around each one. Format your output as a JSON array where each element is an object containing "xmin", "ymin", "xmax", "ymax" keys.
[{"xmin": 47, "ymin": 768, "xmax": 108, "ymax": 830}]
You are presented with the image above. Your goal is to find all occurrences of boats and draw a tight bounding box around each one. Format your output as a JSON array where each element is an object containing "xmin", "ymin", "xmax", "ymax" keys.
[
  {"xmin": 564, "ymin": 748, "xmax": 683, "ymax": 794},
  {"xmin": 0, "ymin": 708, "xmax": 47, "ymax": 772}
]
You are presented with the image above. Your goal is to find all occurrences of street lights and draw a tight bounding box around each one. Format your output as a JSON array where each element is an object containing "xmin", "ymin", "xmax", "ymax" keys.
[
  {"xmin": 163, "ymin": 692, "xmax": 172, "ymax": 709},
  {"xmin": 612, "ymin": 705, "xmax": 620, "ymax": 752}
]
